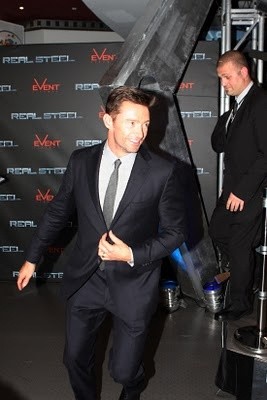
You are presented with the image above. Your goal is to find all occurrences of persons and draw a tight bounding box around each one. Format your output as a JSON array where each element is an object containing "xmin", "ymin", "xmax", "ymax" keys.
[
  {"xmin": 17, "ymin": 86, "xmax": 189, "ymax": 400},
  {"xmin": 209, "ymin": 51, "xmax": 267, "ymax": 321}
]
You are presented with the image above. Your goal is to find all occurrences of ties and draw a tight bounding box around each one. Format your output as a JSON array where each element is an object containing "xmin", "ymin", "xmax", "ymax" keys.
[
  {"xmin": 102, "ymin": 158, "xmax": 122, "ymax": 230},
  {"xmin": 224, "ymin": 99, "xmax": 238, "ymax": 140}
]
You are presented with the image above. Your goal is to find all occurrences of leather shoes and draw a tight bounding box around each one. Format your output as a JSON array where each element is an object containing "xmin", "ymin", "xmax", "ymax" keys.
[
  {"xmin": 252, "ymin": 253, "xmax": 264, "ymax": 295},
  {"xmin": 214, "ymin": 304, "xmax": 252, "ymax": 322}
]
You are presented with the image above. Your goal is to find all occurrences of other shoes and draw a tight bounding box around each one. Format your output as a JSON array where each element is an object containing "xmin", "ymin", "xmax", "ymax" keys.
[{"xmin": 118, "ymin": 385, "xmax": 140, "ymax": 400}]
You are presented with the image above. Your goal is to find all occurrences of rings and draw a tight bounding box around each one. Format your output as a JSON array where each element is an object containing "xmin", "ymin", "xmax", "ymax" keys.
[{"xmin": 102, "ymin": 253, "xmax": 106, "ymax": 259}]
